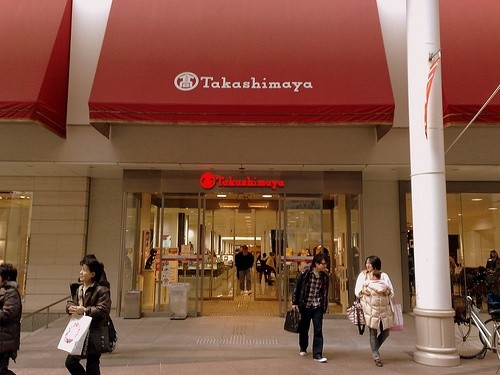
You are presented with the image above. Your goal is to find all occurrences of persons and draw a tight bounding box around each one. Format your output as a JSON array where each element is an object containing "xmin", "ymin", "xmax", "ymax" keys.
[
  {"xmin": 0, "ymin": 262, "xmax": 22, "ymax": 375},
  {"xmin": 363, "ymin": 269, "xmax": 392, "ymax": 293},
  {"xmin": 296, "ymin": 246, "xmax": 331, "ymax": 274},
  {"xmin": 354, "ymin": 255, "xmax": 394, "ymax": 367},
  {"xmin": 232, "ymin": 243, "xmax": 282, "ymax": 295},
  {"xmin": 64, "ymin": 252, "xmax": 112, "ymax": 374},
  {"xmin": 291, "ymin": 253, "xmax": 330, "ymax": 363},
  {"xmin": 464, "ymin": 249, "xmax": 500, "ymax": 315}
]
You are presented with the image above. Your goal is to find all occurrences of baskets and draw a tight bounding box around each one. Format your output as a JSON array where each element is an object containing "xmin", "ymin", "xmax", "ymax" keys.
[{"xmin": 452, "ymin": 295, "xmax": 465, "ymax": 312}]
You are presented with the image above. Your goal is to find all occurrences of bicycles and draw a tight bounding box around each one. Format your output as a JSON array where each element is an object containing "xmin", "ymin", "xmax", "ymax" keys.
[{"xmin": 451, "ymin": 283, "xmax": 500, "ymax": 360}]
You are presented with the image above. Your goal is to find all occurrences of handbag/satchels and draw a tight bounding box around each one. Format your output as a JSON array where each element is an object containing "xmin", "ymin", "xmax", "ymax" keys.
[
  {"xmin": 284, "ymin": 307, "xmax": 302, "ymax": 334},
  {"xmin": 389, "ymin": 298, "xmax": 404, "ymax": 331},
  {"xmin": 56, "ymin": 307, "xmax": 93, "ymax": 356},
  {"xmin": 93, "ymin": 313, "xmax": 118, "ymax": 352},
  {"xmin": 347, "ymin": 295, "xmax": 366, "ymax": 336}
]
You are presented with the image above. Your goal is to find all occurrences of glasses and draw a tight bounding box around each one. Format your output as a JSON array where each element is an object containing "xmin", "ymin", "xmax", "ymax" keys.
[{"xmin": 319, "ymin": 262, "xmax": 328, "ymax": 266}]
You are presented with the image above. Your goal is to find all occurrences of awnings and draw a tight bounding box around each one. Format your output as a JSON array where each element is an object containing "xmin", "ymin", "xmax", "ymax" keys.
[
  {"xmin": 89, "ymin": 0, "xmax": 395, "ymax": 123},
  {"xmin": 0, "ymin": 0, "xmax": 74, "ymax": 142},
  {"xmin": 439, "ymin": 0, "xmax": 499, "ymax": 124}
]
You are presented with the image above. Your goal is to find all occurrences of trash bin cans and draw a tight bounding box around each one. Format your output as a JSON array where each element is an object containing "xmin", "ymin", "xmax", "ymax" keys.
[
  {"xmin": 123, "ymin": 290, "xmax": 142, "ymax": 319},
  {"xmin": 168, "ymin": 283, "xmax": 189, "ymax": 320}
]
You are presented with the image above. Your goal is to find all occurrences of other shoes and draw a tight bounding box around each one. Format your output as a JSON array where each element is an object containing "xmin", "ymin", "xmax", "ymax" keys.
[
  {"xmin": 240, "ymin": 290, "xmax": 244, "ymax": 295},
  {"xmin": 313, "ymin": 357, "xmax": 327, "ymax": 363},
  {"xmin": 247, "ymin": 290, "xmax": 252, "ymax": 295},
  {"xmin": 299, "ymin": 349, "xmax": 307, "ymax": 356},
  {"xmin": 375, "ymin": 358, "xmax": 383, "ymax": 367},
  {"xmin": 268, "ymin": 283, "xmax": 273, "ymax": 286}
]
